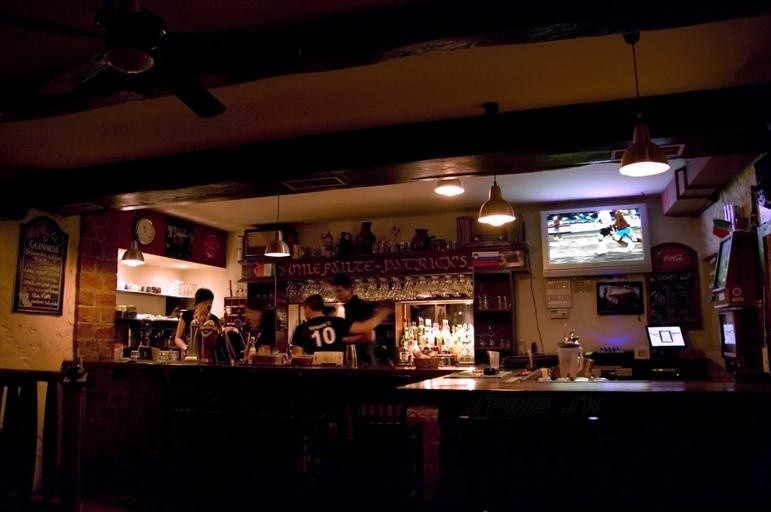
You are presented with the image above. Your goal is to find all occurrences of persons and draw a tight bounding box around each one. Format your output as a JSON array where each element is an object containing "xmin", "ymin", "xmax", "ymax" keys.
[
  {"xmin": 174, "ymin": 288, "xmax": 220, "ymax": 357},
  {"xmin": 289, "ymin": 294, "xmax": 393, "ymax": 357},
  {"xmin": 330, "ymin": 272, "xmax": 373, "ymax": 368},
  {"xmin": 238, "ymin": 302, "xmax": 276, "ymax": 351},
  {"xmin": 553, "ymin": 210, "xmax": 642, "ymax": 247}
]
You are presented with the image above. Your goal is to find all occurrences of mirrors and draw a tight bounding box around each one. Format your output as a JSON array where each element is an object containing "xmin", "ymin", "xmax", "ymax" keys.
[
  {"xmin": 403, "ymin": 305, "xmax": 474, "ymax": 349},
  {"xmin": 298, "ymin": 303, "xmax": 346, "ymax": 328}
]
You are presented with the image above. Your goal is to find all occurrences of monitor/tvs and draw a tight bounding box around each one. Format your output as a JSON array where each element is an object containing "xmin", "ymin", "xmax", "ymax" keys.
[
  {"xmin": 540, "ymin": 203, "xmax": 654, "ymax": 279},
  {"xmin": 645, "ymin": 324, "xmax": 686, "ymax": 349}
]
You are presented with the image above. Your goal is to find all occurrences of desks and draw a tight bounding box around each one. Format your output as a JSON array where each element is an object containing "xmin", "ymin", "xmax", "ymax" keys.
[{"xmin": 397, "ymin": 371, "xmax": 771, "ymax": 512}]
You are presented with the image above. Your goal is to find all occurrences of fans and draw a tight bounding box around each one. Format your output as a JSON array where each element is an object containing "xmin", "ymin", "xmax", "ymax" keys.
[{"xmin": 40, "ymin": 0, "xmax": 226, "ymax": 119}]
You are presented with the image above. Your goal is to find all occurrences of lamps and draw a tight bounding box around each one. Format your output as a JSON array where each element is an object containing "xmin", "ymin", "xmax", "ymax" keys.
[
  {"xmin": 434, "ymin": 177, "xmax": 464, "ymax": 196},
  {"xmin": 618, "ymin": 29, "xmax": 670, "ymax": 177},
  {"xmin": 478, "ymin": 176, "xmax": 516, "ymax": 226},
  {"xmin": 121, "ymin": 209, "xmax": 145, "ymax": 266},
  {"xmin": 264, "ymin": 195, "xmax": 291, "ymax": 258}
]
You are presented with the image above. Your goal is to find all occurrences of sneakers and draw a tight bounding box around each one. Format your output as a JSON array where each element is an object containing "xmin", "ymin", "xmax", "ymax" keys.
[{"xmin": 595, "ymin": 236, "xmax": 642, "ymax": 255}]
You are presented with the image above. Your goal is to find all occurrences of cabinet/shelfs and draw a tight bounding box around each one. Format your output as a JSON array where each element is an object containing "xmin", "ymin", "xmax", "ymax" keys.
[
  {"xmin": 116, "ymin": 287, "xmax": 196, "ymax": 329},
  {"xmin": 237, "ymin": 246, "xmax": 530, "ymax": 366}
]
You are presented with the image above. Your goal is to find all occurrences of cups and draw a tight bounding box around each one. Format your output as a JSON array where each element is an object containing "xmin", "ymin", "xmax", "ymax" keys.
[
  {"xmin": 542, "ymin": 369, "xmax": 547, "ymax": 377},
  {"xmin": 558, "ymin": 343, "xmax": 583, "ymax": 380},
  {"xmin": 130, "ymin": 351, "xmax": 180, "ymax": 362},
  {"xmin": 487, "ymin": 350, "xmax": 500, "ymax": 369}
]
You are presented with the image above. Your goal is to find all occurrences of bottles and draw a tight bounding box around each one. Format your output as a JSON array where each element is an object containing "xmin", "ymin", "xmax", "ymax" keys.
[
  {"xmin": 400, "ymin": 316, "xmax": 474, "ymax": 364},
  {"xmin": 518, "ymin": 338, "xmax": 525, "ymax": 355},
  {"xmin": 248, "ymin": 337, "xmax": 257, "ymax": 365},
  {"xmin": 324, "ymin": 230, "xmax": 333, "ymax": 251},
  {"xmin": 113, "ymin": 343, "xmax": 123, "ymax": 362}
]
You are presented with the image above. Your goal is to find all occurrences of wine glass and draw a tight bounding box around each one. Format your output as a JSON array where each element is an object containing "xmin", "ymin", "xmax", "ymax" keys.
[
  {"xmin": 350, "ymin": 274, "xmax": 474, "ymax": 302},
  {"xmin": 280, "ymin": 278, "xmax": 337, "ymax": 304}
]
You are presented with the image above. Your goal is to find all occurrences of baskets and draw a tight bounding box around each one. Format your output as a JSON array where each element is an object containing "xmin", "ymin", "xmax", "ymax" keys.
[{"xmin": 413, "ymin": 358, "xmax": 439, "ymax": 368}]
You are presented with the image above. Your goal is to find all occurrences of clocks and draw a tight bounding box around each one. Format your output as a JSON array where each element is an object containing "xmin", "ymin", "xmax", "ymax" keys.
[{"xmin": 136, "ymin": 218, "xmax": 156, "ymax": 245}]
